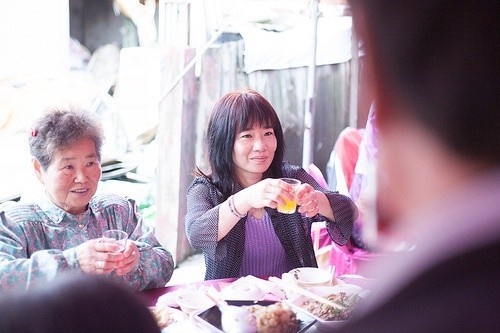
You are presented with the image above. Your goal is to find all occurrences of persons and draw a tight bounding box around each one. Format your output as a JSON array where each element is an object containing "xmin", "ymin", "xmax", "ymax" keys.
[
  {"xmin": 1, "ymin": 109, "xmax": 176, "ymax": 333},
  {"xmin": 315, "ymin": 0, "xmax": 500, "ymax": 333},
  {"xmin": 184, "ymin": 86, "xmax": 359, "ymax": 281}
]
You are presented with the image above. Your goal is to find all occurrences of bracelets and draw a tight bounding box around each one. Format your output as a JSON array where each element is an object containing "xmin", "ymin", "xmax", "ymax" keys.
[{"xmin": 227, "ymin": 196, "xmax": 248, "ymax": 218}]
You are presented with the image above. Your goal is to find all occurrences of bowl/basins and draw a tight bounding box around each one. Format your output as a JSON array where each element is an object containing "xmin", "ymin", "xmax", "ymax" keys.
[{"xmin": 286, "ymin": 267, "xmax": 333, "ymax": 289}]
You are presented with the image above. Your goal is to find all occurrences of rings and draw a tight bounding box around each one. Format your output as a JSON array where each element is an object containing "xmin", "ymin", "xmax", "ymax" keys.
[{"xmin": 99, "ymin": 260, "xmax": 105, "ymax": 268}]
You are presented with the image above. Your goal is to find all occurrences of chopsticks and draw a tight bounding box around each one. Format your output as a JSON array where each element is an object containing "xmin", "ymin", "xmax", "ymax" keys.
[{"xmin": 268, "ymin": 276, "xmax": 344, "ymax": 310}]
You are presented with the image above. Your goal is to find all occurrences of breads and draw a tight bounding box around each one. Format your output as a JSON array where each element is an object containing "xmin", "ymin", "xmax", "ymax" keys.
[{"xmin": 255, "ymin": 303, "xmax": 302, "ymax": 333}]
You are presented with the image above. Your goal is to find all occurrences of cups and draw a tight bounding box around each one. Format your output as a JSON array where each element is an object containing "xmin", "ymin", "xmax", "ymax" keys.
[
  {"xmin": 102, "ymin": 229, "xmax": 128, "ymax": 271},
  {"xmin": 276, "ymin": 177, "xmax": 301, "ymax": 214}
]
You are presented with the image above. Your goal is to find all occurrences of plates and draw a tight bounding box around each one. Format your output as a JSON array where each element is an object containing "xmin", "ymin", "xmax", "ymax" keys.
[
  {"xmin": 193, "ymin": 299, "xmax": 318, "ymax": 333},
  {"xmin": 146, "ymin": 306, "xmax": 195, "ymax": 333},
  {"xmin": 286, "ymin": 287, "xmax": 364, "ymax": 327}
]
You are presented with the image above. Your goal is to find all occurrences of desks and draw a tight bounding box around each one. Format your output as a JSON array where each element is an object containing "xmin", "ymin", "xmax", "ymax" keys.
[{"xmin": 130, "ymin": 275, "xmax": 381, "ymax": 333}]
